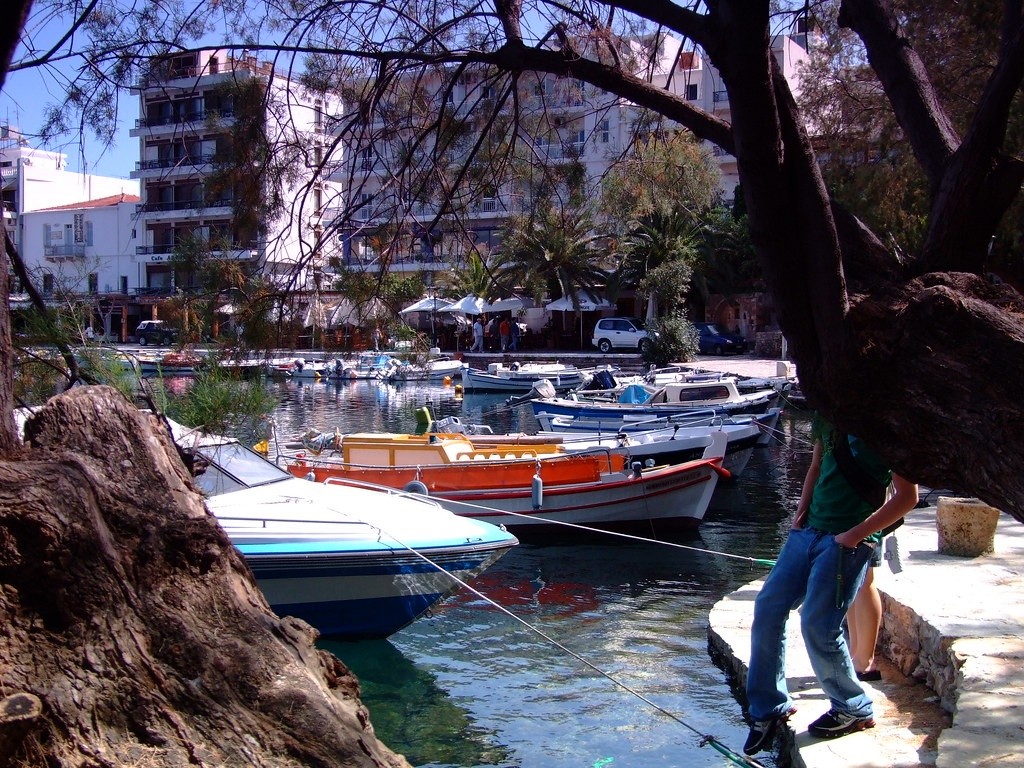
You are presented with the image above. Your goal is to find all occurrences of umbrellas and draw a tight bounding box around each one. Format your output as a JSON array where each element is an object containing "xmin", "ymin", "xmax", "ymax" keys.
[
  {"xmin": 645, "ymin": 294, "xmax": 659, "ymax": 326},
  {"xmin": 438, "ymin": 294, "xmax": 491, "ymax": 336},
  {"xmin": 547, "ymin": 289, "xmax": 618, "ymax": 350},
  {"xmin": 355, "ymin": 295, "xmax": 392, "ymax": 346},
  {"xmin": 397, "ymin": 296, "xmax": 453, "ymax": 336},
  {"xmin": 330, "ymin": 296, "xmax": 359, "ymax": 348},
  {"xmin": 302, "ymin": 298, "xmax": 328, "ymax": 348},
  {"xmin": 214, "ymin": 301, "xmax": 245, "ymax": 327},
  {"xmin": 484, "ymin": 296, "xmax": 534, "ymax": 322},
  {"xmin": 265, "ymin": 298, "xmax": 295, "ymax": 342}
]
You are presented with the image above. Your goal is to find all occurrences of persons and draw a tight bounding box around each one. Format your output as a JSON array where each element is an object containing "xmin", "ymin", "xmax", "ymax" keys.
[
  {"xmin": 470, "ymin": 317, "xmax": 519, "ymax": 353},
  {"xmin": 237, "ymin": 323, "xmax": 247, "ymax": 348},
  {"xmin": 370, "ymin": 323, "xmax": 392, "ymax": 352},
  {"xmin": 82, "ymin": 324, "xmax": 95, "ymax": 343},
  {"xmin": 743, "ymin": 409, "xmax": 918, "ymax": 756}
]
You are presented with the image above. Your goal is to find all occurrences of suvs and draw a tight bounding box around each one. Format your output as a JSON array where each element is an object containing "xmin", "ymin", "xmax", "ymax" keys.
[
  {"xmin": 591, "ymin": 317, "xmax": 659, "ymax": 353},
  {"xmin": 135, "ymin": 320, "xmax": 213, "ymax": 346},
  {"xmin": 692, "ymin": 322, "xmax": 748, "ymax": 355}
]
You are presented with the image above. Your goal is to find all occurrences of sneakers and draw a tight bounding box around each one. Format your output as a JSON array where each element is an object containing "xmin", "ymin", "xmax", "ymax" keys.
[
  {"xmin": 808, "ymin": 708, "xmax": 876, "ymax": 738},
  {"xmin": 743, "ymin": 705, "xmax": 799, "ymax": 756}
]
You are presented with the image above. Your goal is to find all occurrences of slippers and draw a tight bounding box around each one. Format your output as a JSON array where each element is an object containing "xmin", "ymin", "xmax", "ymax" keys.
[{"xmin": 854, "ymin": 670, "xmax": 881, "ymax": 681}]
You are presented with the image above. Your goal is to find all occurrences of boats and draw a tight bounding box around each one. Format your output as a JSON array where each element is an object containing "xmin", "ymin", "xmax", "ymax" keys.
[
  {"xmin": 260, "ymin": 427, "xmax": 731, "ymax": 534},
  {"xmin": 94, "ymin": 344, "xmax": 803, "ymax": 481},
  {"xmin": 139, "ymin": 404, "xmax": 521, "ymax": 643}
]
